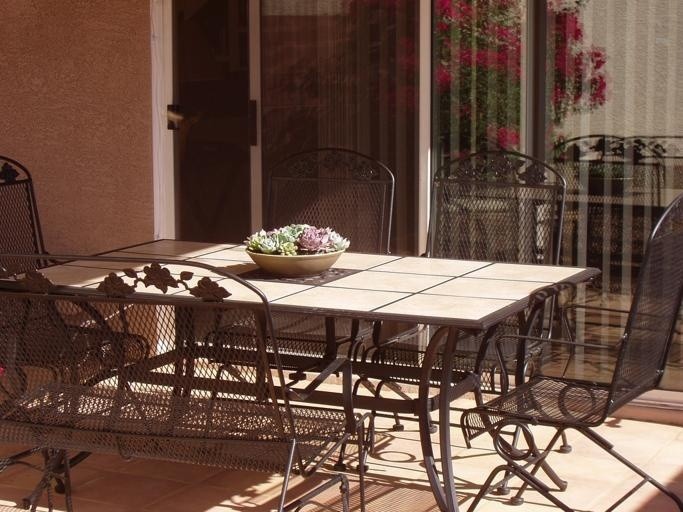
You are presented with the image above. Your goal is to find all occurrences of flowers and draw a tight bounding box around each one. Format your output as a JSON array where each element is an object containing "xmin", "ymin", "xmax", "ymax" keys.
[
  {"xmin": 392, "ymin": 1, "xmax": 610, "ymax": 150},
  {"xmin": 243, "ymin": 221, "xmax": 350, "ymax": 254}
]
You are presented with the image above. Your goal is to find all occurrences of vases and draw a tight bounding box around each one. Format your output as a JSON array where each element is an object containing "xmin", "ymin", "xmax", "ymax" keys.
[{"xmin": 246, "ymin": 249, "xmax": 346, "ymax": 277}]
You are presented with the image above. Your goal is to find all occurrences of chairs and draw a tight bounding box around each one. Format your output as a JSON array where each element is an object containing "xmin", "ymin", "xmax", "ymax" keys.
[
  {"xmin": 351, "ymin": 149, "xmax": 568, "ymax": 474},
  {"xmin": 548, "ymin": 136, "xmax": 682, "ymax": 295},
  {"xmin": 459, "ymin": 191, "xmax": 683, "ymax": 507},
  {"xmin": 156, "ymin": 151, "xmax": 397, "ymax": 433},
  {"xmin": 2, "ymin": 253, "xmax": 369, "ymax": 512},
  {"xmin": 2, "ymin": 149, "xmax": 148, "ymax": 420}
]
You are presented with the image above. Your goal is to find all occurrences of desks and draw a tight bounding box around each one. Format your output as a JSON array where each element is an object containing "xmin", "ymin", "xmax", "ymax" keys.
[{"xmin": 4, "ymin": 236, "xmax": 606, "ymax": 509}]
